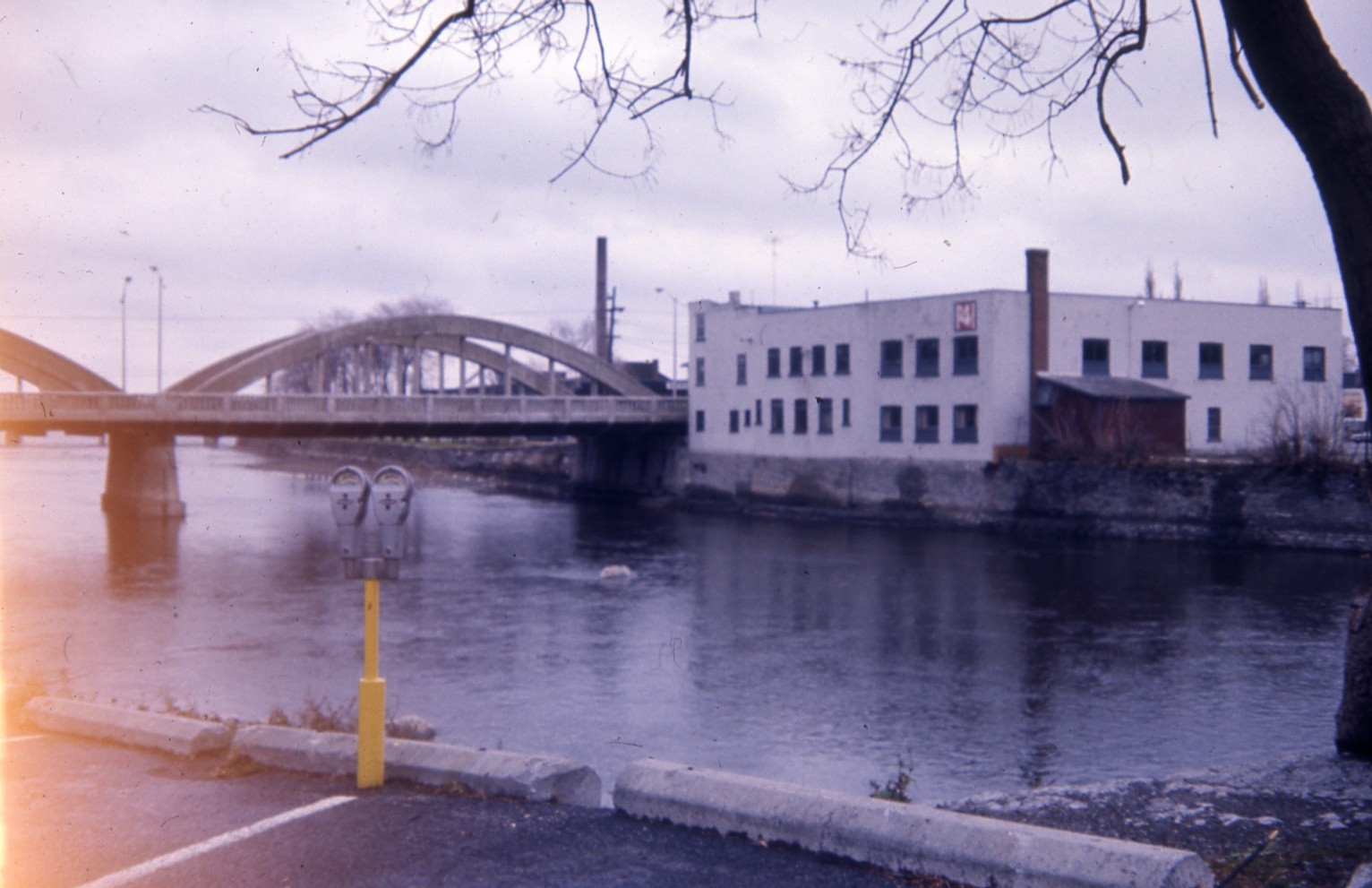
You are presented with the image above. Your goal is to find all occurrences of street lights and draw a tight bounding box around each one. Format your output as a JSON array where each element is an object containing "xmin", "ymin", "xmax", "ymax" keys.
[
  {"xmin": 150, "ymin": 264, "xmax": 168, "ymax": 392},
  {"xmin": 117, "ymin": 271, "xmax": 134, "ymax": 390}
]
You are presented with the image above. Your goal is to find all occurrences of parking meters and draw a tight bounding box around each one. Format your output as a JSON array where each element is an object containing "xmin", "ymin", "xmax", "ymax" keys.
[{"xmin": 327, "ymin": 461, "xmax": 415, "ymax": 789}]
